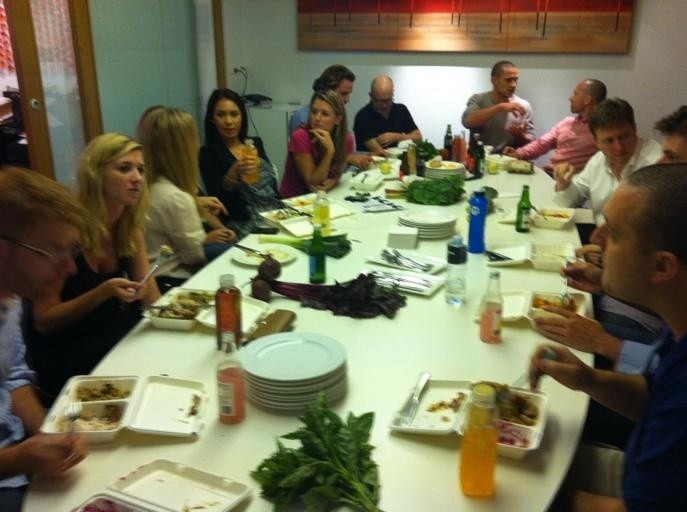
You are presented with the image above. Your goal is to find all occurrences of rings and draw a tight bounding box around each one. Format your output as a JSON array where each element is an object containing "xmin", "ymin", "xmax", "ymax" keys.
[{"xmin": 597, "ymin": 258, "xmax": 601, "ymax": 263}]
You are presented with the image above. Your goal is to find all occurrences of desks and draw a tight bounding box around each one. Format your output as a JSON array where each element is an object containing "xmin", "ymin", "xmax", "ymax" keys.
[{"xmin": 246, "ymin": 99, "xmax": 304, "ymax": 151}]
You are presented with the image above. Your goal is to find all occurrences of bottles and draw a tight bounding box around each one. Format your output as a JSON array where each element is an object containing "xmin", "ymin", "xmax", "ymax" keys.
[
  {"xmin": 457, "ymin": 383, "xmax": 500, "ymax": 497},
  {"xmin": 239, "ymin": 138, "xmax": 261, "ymax": 185},
  {"xmin": 216, "ymin": 332, "xmax": 245, "ymax": 425},
  {"xmin": 313, "ymin": 190, "xmax": 331, "ymax": 238},
  {"xmin": 514, "ymin": 185, "xmax": 531, "ymax": 232},
  {"xmin": 444, "ymin": 235, "xmax": 467, "ymax": 306},
  {"xmin": 213, "ymin": 274, "xmax": 242, "ymax": 351},
  {"xmin": 307, "ymin": 224, "xmax": 326, "ymax": 283},
  {"xmin": 397, "ymin": 143, "xmax": 424, "ymax": 182},
  {"xmin": 478, "ymin": 271, "xmax": 504, "ymax": 345},
  {"xmin": 442, "ymin": 123, "xmax": 485, "ymax": 177},
  {"xmin": 466, "ymin": 189, "xmax": 486, "ymax": 253}
]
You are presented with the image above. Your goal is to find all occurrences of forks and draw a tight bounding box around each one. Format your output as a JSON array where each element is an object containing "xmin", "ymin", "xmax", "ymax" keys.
[
  {"xmin": 136, "ymin": 253, "xmax": 171, "ymax": 289},
  {"xmin": 63, "ymin": 398, "xmax": 82, "ymax": 439}
]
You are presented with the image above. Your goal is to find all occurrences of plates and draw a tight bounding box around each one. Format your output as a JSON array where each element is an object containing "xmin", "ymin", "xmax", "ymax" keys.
[
  {"xmin": 373, "ymin": 156, "xmax": 402, "ymax": 179},
  {"xmin": 233, "ymin": 243, "xmax": 298, "ymax": 265},
  {"xmin": 424, "ymin": 160, "xmax": 466, "ymax": 182},
  {"xmin": 361, "ymin": 199, "xmax": 403, "ymax": 212},
  {"xmin": 360, "ymin": 269, "xmax": 447, "ymax": 297},
  {"xmin": 367, "ymin": 248, "xmax": 447, "ymax": 274},
  {"xmin": 486, "ymin": 153, "xmax": 517, "ymax": 168},
  {"xmin": 396, "ymin": 207, "xmax": 457, "ymax": 239},
  {"xmin": 236, "ymin": 333, "xmax": 349, "ymax": 411}
]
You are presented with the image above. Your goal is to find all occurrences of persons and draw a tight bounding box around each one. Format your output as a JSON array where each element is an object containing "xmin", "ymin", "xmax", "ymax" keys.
[
  {"xmin": 137, "ymin": 106, "xmax": 236, "ymax": 287},
  {"xmin": 532, "ymin": 104, "xmax": 686, "ymax": 442},
  {"xmin": 288, "ymin": 64, "xmax": 373, "ymax": 173},
  {"xmin": 461, "ymin": 60, "xmax": 536, "ymax": 156},
  {"xmin": 1, "ymin": 167, "xmax": 92, "ymax": 511},
  {"xmin": 354, "ymin": 75, "xmax": 422, "ymax": 157},
  {"xmin": 553, "ymin": 97, "xmax": 664, "ymax": 266},
  {"xmin": 278, "ymin": 89, "xmax": 353, "ymax": 199},
  {"xmin": 199, "ymin": 88, "xmax": 286, "ymax": 239},
  {"xmin": 527, "ymin": 161, "xmax": 686, "ymax": 512},
  {"xmin": 503, "ymin": 78, "xmax": 606, "ymax": 180},
  {"xmin": 35, "ymin": 132, "xmax": 162, "ymax": 377}
]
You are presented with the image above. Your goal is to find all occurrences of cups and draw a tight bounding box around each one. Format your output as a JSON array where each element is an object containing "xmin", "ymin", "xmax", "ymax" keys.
[{"xmin": 486, "ymin": 155, "xmax": 500, "ymax": 175}]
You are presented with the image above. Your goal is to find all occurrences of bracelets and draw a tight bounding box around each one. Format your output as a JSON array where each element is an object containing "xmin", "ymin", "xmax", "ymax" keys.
[{"xmin": 225, "ymin": 173, "xmax": 237, "ymax": 183}]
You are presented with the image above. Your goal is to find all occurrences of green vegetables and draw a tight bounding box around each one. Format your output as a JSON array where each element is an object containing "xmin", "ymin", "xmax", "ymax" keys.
[{"xmin": 249, "ymin": 389, "xmax": 384, "ymax": 512}]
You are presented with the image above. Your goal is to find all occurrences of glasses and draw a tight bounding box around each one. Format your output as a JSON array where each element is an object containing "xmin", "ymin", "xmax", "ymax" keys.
[{"xmin": 0, "ymin": 233, "xmax": 79, "ymax": 265}]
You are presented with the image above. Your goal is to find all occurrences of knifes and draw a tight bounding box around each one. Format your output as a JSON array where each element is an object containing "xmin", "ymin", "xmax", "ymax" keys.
[
  {"xmin": 379, "ymin": 249, "xmax": 431, "ymax": 271},
  {"xmin": 227, "ymin": 242, "xmax": 261, "ymax": 255},
  {"xmin": 369, "ymin": 271, "xmax": 432, "ymax": 293}
]
